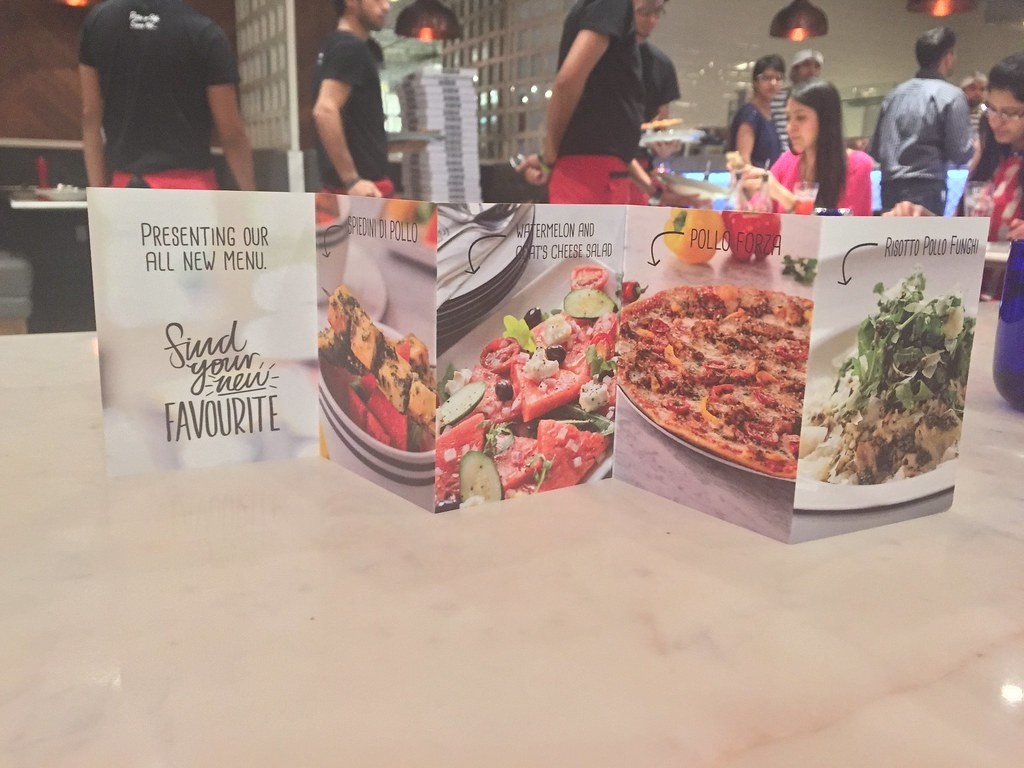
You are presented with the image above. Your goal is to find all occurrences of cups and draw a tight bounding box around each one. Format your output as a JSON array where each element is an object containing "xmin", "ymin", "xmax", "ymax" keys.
[
  {"xmin": 963, "ymin": 180, "xmax": 994, "ymax": 217},
  {"xmin": 992, "ymin": 239, "xmax": 1024, "ymax": 411},
  {"xmin": 792, "ymin": 182, "xmax": 819, "ymax": 215}
]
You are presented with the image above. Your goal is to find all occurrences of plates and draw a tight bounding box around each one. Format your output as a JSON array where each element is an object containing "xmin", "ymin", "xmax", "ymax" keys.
[
  {"xmin": 661, "ymin": 171, "xmax": 731, "ymax": 199},
  {"xmin": 437, "ymin": 244, "xmax": 620, "ymax": 513},
  {"xmin": 386, "ymin": 131, "xmax": 445, "ymax": 143},
  {"xmin": 37, "ymin": 188, "xmax": 87, "ymax": 200},
  {"xmin": 638, "ymin": 129, "xmax": 706, "ymax": 147},
  {"xmin": 618, "ymin": 280, "xmax": 814, "ymax": 482},
  {"xmin": 437, "ymin": 203, "xmax": 535, "ymax": 341},
  {"xmin": 316, "ymin": 240, "xmax": 387, "ymax": 328},
  {"xmin": 792, "ymin": 313, "xmax": 957, "ymax": 511}
]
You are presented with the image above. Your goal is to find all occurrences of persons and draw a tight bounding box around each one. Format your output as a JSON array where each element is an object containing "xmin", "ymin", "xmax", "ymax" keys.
[
  {"xmin": 310, "ymin": 0, "xmax": 403, "ymax": 198},
  {"xmin": 951, "ymin": 70, "xmax": 990, "ymax": 165},
  {"xmin": 723, "ymin": 77, "xmax": 875, "ymax": 215},
  {"xmin": 767, "ymin": 46, "xmax": 822, "ymax": 155},
  {"xmin": 863, "ymin": 22, "xmax": 979, "ymax": 218},
  {"xmin": 76, "ymin": 1, "xmax": 258, "ymax": 194},
  {"xmin": 872, "ymin": 47, "xmax": 1023, "ymax": 299},
  {"xmin": 512, "ymin": 0, "xmax": 649, "ymax": 205},
  {"xmin": 624, "ymin": 0, "xmax": 681, "ymax": 178},
  {"xmin": 729, "ymin": 54, "xmax": 782, "ymax": 196}
]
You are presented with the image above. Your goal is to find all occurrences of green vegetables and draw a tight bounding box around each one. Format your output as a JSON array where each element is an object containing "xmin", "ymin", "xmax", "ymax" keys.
[{"xmin": 830, "ymin": 266, "xmax": 977, "ymax": 415}]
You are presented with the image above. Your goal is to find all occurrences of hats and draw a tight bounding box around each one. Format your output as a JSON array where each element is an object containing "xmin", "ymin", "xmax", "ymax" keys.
[
  {"xmin": 791, "ymin": 49, "xmax": 824, "ymax": 65},
  {"xmin": 959, "ymin": 73, "xmax": 990, "ymax": 90}
]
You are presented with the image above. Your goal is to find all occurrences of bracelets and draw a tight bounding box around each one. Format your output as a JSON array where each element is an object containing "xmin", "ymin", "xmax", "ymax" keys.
[
  {"xmin": 342, "ymin": 174, "xmax": 363, "ymax": 193},
  {"xmin": 534, "ymin": 149, "xmax": 554, "ymax": 177}
]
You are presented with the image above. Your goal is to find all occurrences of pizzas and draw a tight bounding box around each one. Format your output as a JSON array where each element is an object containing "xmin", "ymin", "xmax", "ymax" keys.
[{"xmin": 619, "ymin": 284, "xmax": 815, "ymax": 480}]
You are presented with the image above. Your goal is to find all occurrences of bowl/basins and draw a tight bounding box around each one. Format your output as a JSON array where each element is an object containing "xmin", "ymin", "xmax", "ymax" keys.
[
  {"xmin": 316, "ymin": 195, "xmax": 350, "ymax": 304},
  {"xmin": 319, "ymin": 322, "xmax": 436, "ymax": 516}
]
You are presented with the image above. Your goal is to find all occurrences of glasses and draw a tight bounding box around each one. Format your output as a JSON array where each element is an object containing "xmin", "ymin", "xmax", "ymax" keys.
[
  {"xmin": 635, "ymin": 7, "xmax": 666, "ymax": 17},
  {"xmin": 756, "ymin": 73, "xmax": 784, "ymax": 81},
  {"xmin": 982, "ymin": 105, "xmax": 1024, "ymax": 120}
]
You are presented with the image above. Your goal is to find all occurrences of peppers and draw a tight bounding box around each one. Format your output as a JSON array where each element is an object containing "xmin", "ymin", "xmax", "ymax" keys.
[{"xmin": 662, "ymin": 209, "xmax": 781, "ymax": 263}]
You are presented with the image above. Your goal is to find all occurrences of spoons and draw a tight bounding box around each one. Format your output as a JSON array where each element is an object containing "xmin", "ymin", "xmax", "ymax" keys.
[{"xmin": 437, "ymin": 203, "xmax": 521, "ymax": 253}]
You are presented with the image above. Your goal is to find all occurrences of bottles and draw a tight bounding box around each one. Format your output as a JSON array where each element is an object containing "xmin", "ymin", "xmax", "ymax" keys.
[
  {"xmin": 724, "ymin": 168, "xmax": 751, "ymax": 211},
  {"xmin": 750, "ymin": 158, "xmax": 773, "ymax": 212}
]
